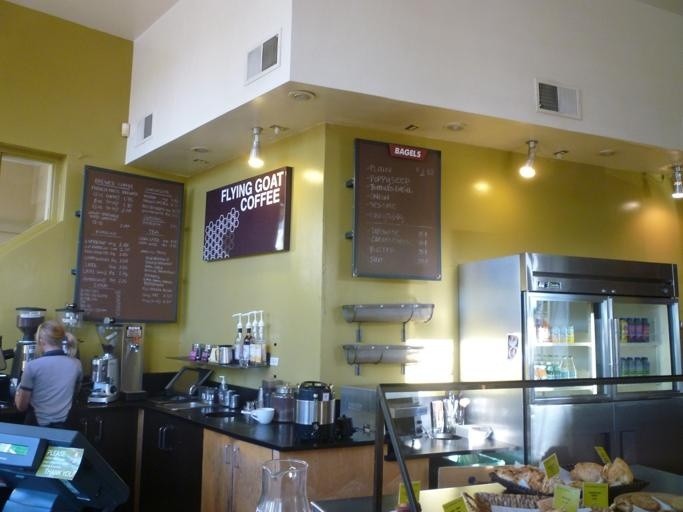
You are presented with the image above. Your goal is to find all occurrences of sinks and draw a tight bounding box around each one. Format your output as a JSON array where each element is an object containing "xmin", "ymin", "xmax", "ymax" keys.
[{"xmin": 161, "ymin": 401, "xmax": 211, "ymax": 410}]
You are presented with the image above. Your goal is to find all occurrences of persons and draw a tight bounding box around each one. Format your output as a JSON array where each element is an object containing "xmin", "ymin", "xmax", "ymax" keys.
[{"xmin": 15, "ymin": 321, "xmax": 83, "ymax": 427}]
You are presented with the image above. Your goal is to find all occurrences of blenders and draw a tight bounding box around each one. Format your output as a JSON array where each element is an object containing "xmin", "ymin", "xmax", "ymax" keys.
[
  {"xmin": 7, "ymin": 306, "xmax": 48, "ymax": 397},
  {"xmin": 86, "ymin": 316, "xmax": 123, "ymax": 404}
]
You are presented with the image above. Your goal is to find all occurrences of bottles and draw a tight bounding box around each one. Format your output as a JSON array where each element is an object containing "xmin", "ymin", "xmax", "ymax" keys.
[
  {"xmin": 255, "ymin": 457, "xmax": 312, "ymax": 512},
  {"xmin": 218, "ymin": 345, "xmax": 233, "ymax": 365},
  {"xmin": 620, "ymin": 317, "xmax": 650, "ymax": 343},
  {"xmin": 271, "ymin": 385, "xmax": 297, "ymax": 424},
  {"xmin": 533, "ymin": 353, "xmax": 577, "ymax": 380},
  {"xmin": 620, "ymin": 357, "xmax": 650, "ymax": 377}
]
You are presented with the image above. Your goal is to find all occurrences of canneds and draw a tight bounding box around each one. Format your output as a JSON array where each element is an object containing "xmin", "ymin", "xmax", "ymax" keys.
[
  {"xmin": 551, "ymin": 327, "xmax": 560, "ymax": 343},
  {"xmin": 565, "ymin": 326, "xmax": 574, "ymax": 344}
]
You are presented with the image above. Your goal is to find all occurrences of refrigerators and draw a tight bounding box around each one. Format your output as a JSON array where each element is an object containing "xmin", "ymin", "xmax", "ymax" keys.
[{"xmin": 456, "ymin": 251, "xmax": 683, "ymax": 476}]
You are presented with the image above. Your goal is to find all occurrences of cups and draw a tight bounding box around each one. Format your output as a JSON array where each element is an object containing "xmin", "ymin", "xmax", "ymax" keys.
[{"xmin": 250, "ymin": 407, "xmax": 275, "ymax": 425}]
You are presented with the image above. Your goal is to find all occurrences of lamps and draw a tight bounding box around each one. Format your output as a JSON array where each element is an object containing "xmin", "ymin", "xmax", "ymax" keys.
[
  {"xmin": 519, "ymin": 141, "xmax": 539, "ymax": 179},
  {"xmin": 246, "ymin": 126, "xmax": 264, "ymax": 167},
  {"xmin": 668, "ymin": 165, "xmax": 683, "ymax": 198}
]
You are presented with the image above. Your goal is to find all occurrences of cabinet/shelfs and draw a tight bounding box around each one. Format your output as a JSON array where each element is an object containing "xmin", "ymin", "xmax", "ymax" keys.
[{"xmin": 69, "ymin": 407, "xmax": 429, "ymax": 512}]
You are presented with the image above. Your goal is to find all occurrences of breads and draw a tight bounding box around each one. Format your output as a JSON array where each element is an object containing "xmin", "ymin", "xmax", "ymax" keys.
[{"xmin": 473, "ymin": 456, "xmax": 683, "ymax": 512}]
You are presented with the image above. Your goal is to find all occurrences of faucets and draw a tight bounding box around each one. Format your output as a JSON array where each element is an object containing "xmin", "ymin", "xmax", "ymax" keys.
[{"xmin": 189, "ymin": 384, "xmax": 218, "ymax": 396}]
